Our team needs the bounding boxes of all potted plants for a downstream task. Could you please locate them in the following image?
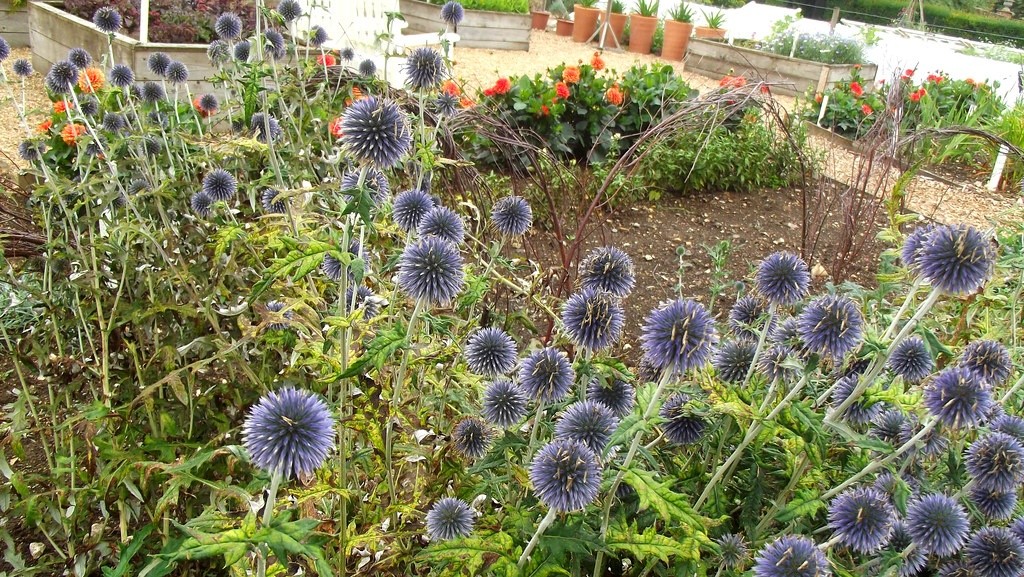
[661,0,695,61]
[694,8,727,41]
[628,0,659,54]
[397,0,531,53]
[573,0,601,44]
[598,0,629,48]
[28,0,340,136]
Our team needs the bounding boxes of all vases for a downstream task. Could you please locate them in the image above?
[531,10,550,31]
[556,19,574,38]
[682,36,879,104]
